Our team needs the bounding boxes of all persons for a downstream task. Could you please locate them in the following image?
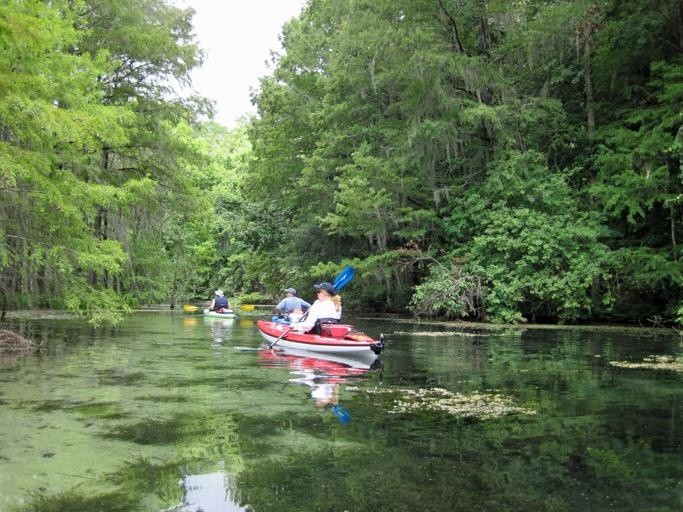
[209,290,231,311]
[295,373,333,408]
[274,282,342,334]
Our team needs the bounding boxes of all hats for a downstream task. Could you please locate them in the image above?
[284,287,297,295]
[313,282,337,295]
[215,290,224,297]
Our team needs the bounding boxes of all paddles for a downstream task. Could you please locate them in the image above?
[267,266,356,349]
[184,304,255,312]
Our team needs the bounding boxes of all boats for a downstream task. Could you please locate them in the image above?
[252,318,385,371]
[203,309,235,319]
[256,341,374,383]
